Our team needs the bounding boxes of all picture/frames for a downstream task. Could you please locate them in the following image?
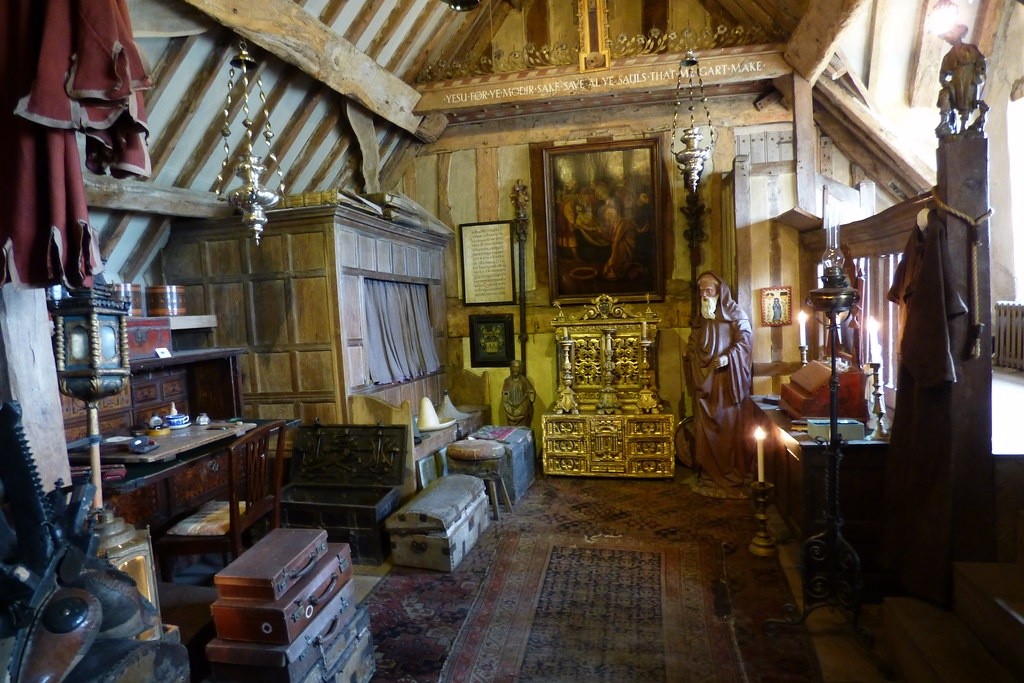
[542,137,665,306]
[468,313,515,368]
[460,220,517,306]
[761,286,792,326]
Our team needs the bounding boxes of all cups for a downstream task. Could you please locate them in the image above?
[166,414,189,425]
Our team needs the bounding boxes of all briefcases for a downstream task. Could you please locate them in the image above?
[206,527,376,683]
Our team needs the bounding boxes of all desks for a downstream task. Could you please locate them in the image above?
[750,394,890,546]
[68,418,300,524]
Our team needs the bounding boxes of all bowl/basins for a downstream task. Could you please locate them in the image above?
[145,286,186,316]
[108,284,142,316]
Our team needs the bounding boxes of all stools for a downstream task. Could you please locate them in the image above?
[447,455,513,521]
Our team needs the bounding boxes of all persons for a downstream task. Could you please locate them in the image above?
[682,271,753,488]
[502,360,536,426]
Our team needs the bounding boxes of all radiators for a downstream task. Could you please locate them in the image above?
[993,300,1024,371]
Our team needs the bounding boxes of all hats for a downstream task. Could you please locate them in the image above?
[436,390,474,420]
[414,397,456,431]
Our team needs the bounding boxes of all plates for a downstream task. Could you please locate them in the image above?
[170,421,191,429]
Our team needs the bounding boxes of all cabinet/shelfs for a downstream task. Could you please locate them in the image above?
[59,315,248,441]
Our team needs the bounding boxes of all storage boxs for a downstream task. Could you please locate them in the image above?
[279,417,408,566]
[807,418,865,440]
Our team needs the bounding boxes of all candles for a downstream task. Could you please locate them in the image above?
[751,425,767,482]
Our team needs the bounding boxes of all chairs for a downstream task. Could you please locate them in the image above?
[160,420,287,559]
